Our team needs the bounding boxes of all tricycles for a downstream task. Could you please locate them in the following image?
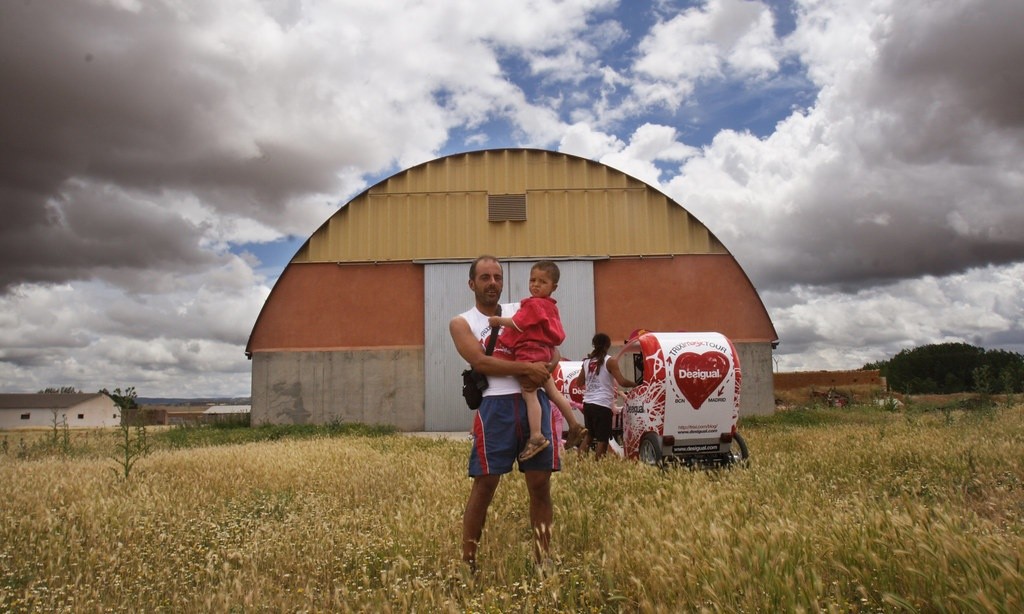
[604,332,750,474]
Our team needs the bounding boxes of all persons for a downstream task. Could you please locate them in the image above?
[576,333,637,461]
[449,256,566,579]
[488,260,588,461]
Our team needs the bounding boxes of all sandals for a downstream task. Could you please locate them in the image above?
[518,435,551,462]
[564,424,588,450]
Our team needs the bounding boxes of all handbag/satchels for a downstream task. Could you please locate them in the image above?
[461,368,489,411]
